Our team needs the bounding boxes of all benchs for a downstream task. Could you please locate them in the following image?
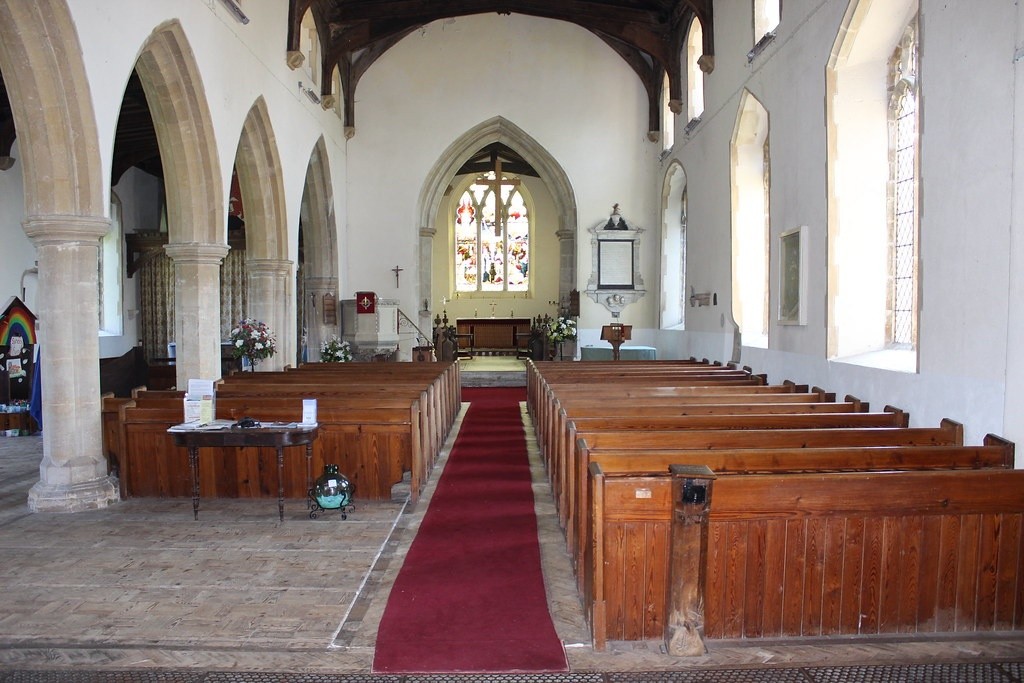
[526,357,1024,647]
[102,358,463,510]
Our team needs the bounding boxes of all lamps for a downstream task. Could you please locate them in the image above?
[746,31,777,63]
[683,116,701,135]
[303,87,321,105]
[221,0,250,25]
[656,148,671,163]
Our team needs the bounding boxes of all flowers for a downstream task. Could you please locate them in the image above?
[318,334,353,363]
[230,317,278,371]
[545,317,578,345]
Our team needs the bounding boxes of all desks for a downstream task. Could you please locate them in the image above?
[168,421,324,523]
[455,333,473,358]
[456,317,532,349]
[580,346,657,360]
[516,332,536,359]
